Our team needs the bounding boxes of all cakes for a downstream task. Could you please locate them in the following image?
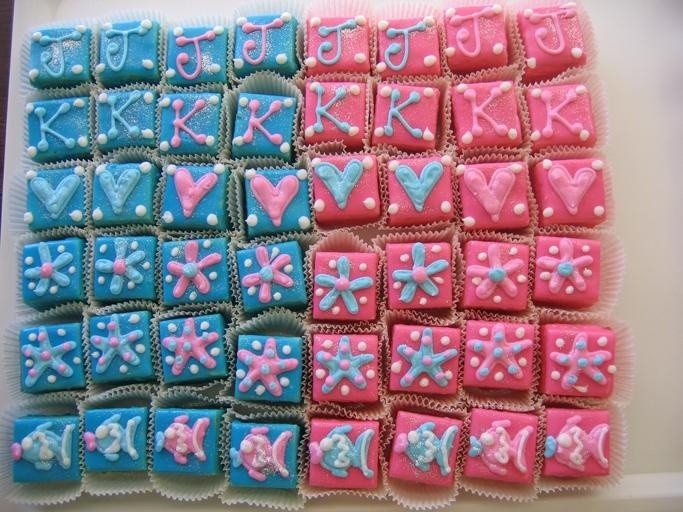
[456,161,530,232]
[23,165,86,232]
[93,235,158,303]
[91,161,159,226]
[459,240,530,314]
[450,79,523,152]
[89,310,156,385]
[84,406,148,472]
[464,319,535,390]
[158,92,222,156]
[231,92,297,162]
[523,85,597,153]
[234,333,304,404]
[303,81,367,148]
[158,314,228,386]
[235,240,309,313]
[160,163,230,232]
[312,332,379,404]
[162,236,231,306]
[18,322,86,394]
[303,15,371,76]
[22,237,86,312]
[444,4,509,73]
[518,2,587,84]
[311,154,380,225]
[165,24,229,86]
[531,158,608,226]
[233,12,299,77]
[152,408,225,476]
[95,18,159,87]
[534,235,601,308]
[375,15,441,79]
[307,418,380,490]
[243,167,311,239]
[96,89,157,154]
[27,24,91,87]
[386,241,453,310]
[229,420,300,489]
[25,94,90,164]
[386,154,454,226]
[387,411,463,488]
[389,323,462,396]
[539,323,618,399]
[10,414,81,483]
[371,82,441,151]
[312,251,379,322]
[465,408,539,484]
[542,406,611,478]
